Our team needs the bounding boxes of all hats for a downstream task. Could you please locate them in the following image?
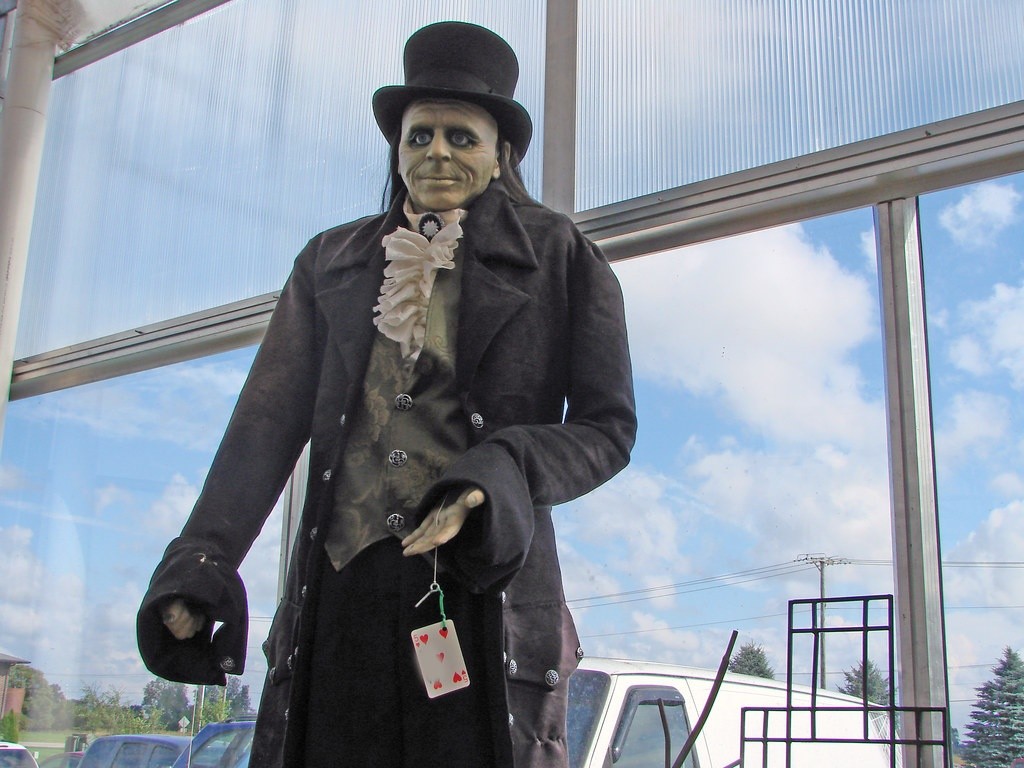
[372,22,533,168]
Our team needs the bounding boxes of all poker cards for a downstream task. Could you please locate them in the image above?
[410,619,471,699]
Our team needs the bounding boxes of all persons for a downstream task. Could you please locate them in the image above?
[136,21,638,768]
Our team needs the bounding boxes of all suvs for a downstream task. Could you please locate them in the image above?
[171,716,255,768]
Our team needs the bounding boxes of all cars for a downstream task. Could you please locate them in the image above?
[38,752,82,768]
[0,740,38,768]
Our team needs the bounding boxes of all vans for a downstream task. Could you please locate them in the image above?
[79,734,237,768]
[566,653,903,768]
